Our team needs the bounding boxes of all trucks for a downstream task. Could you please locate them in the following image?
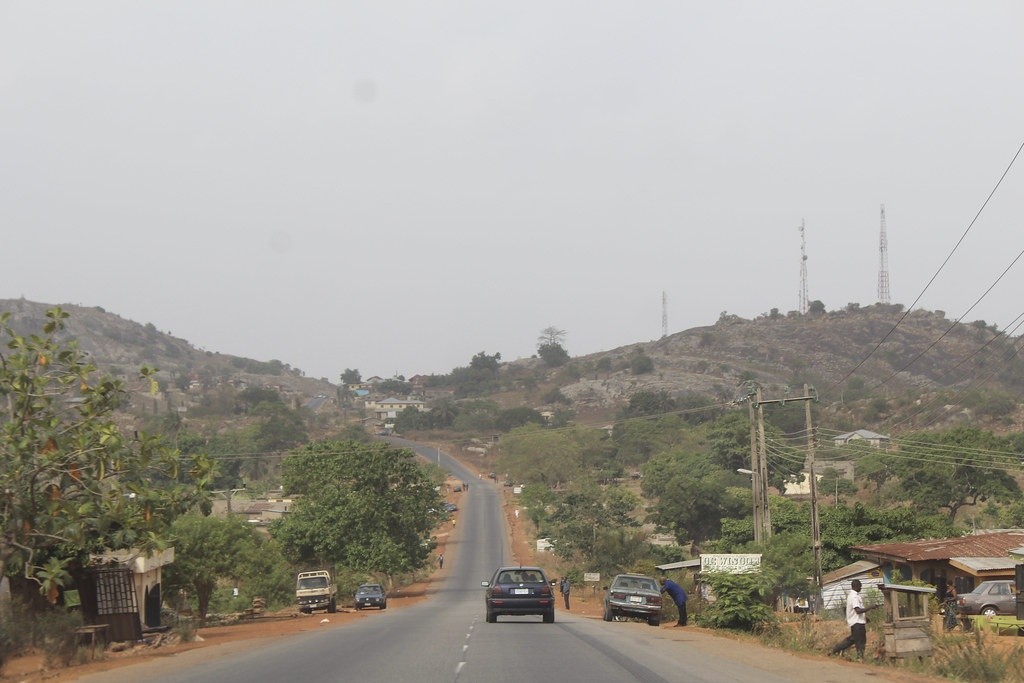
[296,570,339,614]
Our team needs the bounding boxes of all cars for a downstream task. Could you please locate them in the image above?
[481,566,558,624]
[602,574,663,627]
[354,584,387,610]
[956,580,1017,615]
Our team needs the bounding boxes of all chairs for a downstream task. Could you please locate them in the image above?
[529,574,537,580]
[505,578,514,583]
[620,581,628,586]
[641,583,651,589]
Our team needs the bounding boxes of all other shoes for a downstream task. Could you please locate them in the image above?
[674,621,686,627]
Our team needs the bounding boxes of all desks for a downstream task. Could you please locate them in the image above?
[76,623,110,660]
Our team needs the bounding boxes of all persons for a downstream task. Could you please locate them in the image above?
[943,580,957,630]
[660,577,688,627]
[452,519,456,527]
[479,473,481,479]
[831,580,877,660]
[462,483,468,491]
[560,577,570,610]
[437,554,443,569]
[521,573,528,581]
[494,473,496,481]
[515,509,519,518]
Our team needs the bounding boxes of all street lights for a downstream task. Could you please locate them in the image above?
[737,469,771,536]
